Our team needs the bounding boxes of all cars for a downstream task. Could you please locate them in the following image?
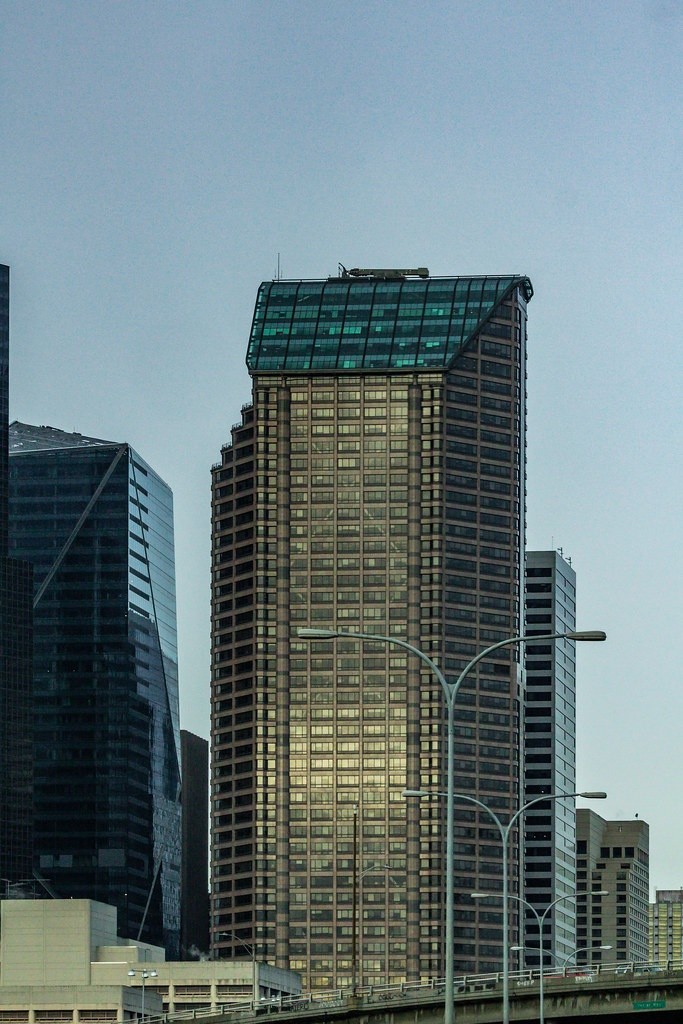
[616,963,662,974]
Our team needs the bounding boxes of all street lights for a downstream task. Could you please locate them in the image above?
[400,788,609,1024]
[470,890,610,1024]
[217,931,256,1001]
[350,803,396,996]
[296,624,610,1024]
[128,968,159,1022]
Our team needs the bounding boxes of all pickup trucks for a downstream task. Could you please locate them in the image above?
[543,966,595,979]
